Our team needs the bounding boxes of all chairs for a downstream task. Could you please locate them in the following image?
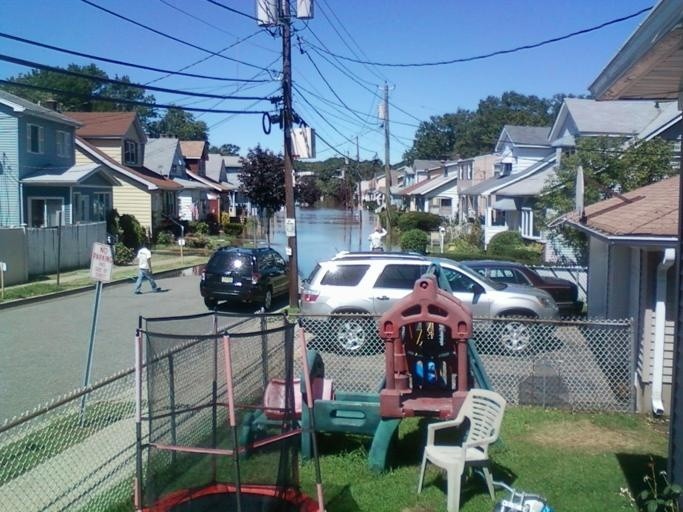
[417,387,507,512]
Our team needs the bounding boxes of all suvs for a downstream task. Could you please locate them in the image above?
[297,252,562,357]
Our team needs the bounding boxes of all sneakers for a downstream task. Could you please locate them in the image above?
[157,288,161,292]
[134,292,142,294]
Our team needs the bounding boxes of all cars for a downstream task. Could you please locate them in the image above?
[199,246,295,311]
[459,260,585,314]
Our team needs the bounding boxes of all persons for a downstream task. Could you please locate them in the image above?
[132,241,160,293]
[367,225,388,254]
